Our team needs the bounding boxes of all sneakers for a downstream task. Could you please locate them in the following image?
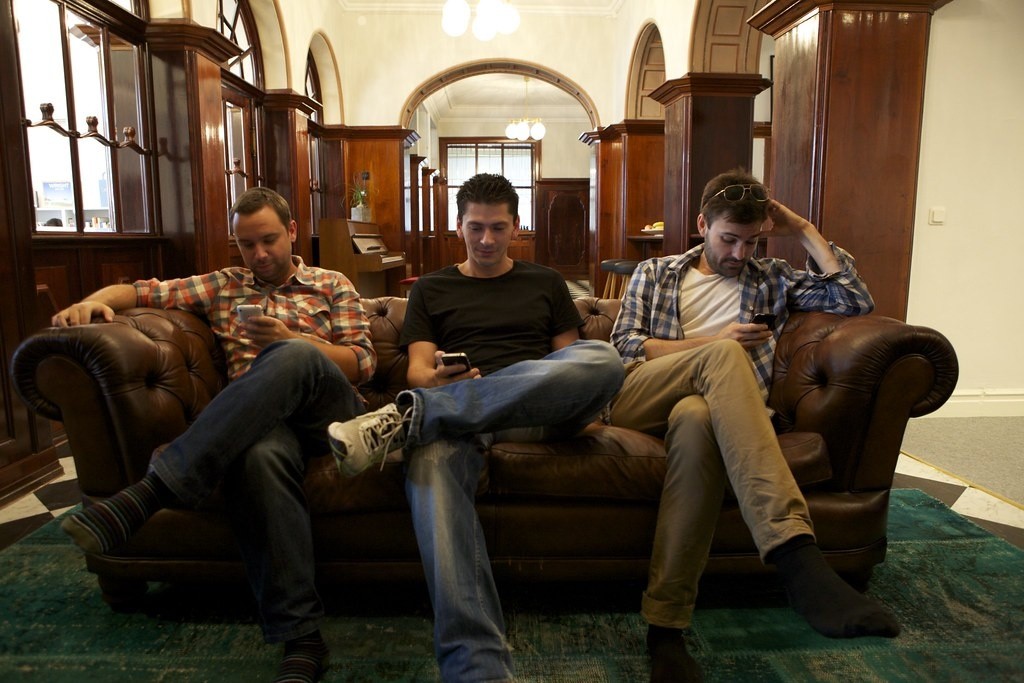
[327,402,404,481]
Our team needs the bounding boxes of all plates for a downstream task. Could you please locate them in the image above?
[641,229,664,236]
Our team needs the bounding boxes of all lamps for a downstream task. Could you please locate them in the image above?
[440,0,521,42]
[504,76,546,141]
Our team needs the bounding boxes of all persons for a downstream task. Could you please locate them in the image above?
[50,187,378,682]
[597,166,901,682]
[327,171,626,683]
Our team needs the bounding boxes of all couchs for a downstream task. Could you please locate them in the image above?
[10,294,960,639]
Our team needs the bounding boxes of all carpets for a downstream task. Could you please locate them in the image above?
[0,483,1024,682]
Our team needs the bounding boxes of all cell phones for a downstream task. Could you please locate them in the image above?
[750,313,777,330]
[237,305,264,323]
[442,353,471,377]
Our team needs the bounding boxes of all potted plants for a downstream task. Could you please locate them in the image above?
[338,170,372,223]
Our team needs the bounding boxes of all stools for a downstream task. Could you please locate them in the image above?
[600,259,629,299]
[612,260,639,299]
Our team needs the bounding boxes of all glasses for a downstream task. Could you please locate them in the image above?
[700,183,770,212]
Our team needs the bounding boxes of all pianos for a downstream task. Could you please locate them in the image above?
[318,216,405,299]
